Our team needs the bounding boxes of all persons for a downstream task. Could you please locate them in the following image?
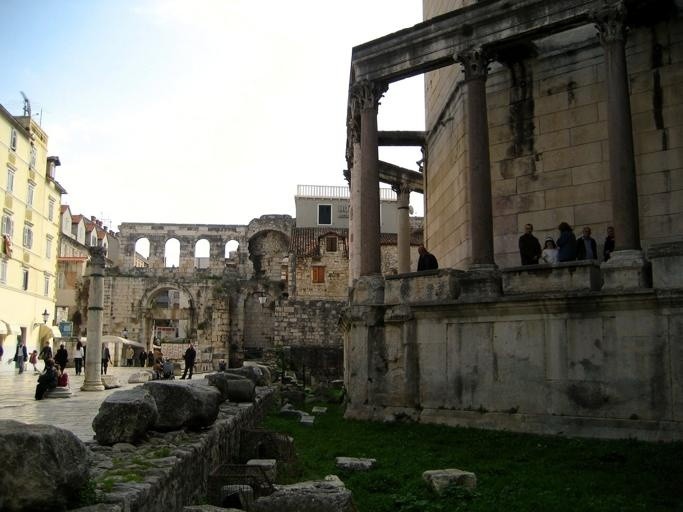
[0,340,174,400]
[576,227,597,260]
[518,223,542,264]
[541,236,559,263]
[555,222,578,261]
[415,246,438,270]
[601,226,616,260]
[179,343,195,380]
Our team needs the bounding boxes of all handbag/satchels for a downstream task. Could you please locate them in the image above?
[57,374,67,387]
